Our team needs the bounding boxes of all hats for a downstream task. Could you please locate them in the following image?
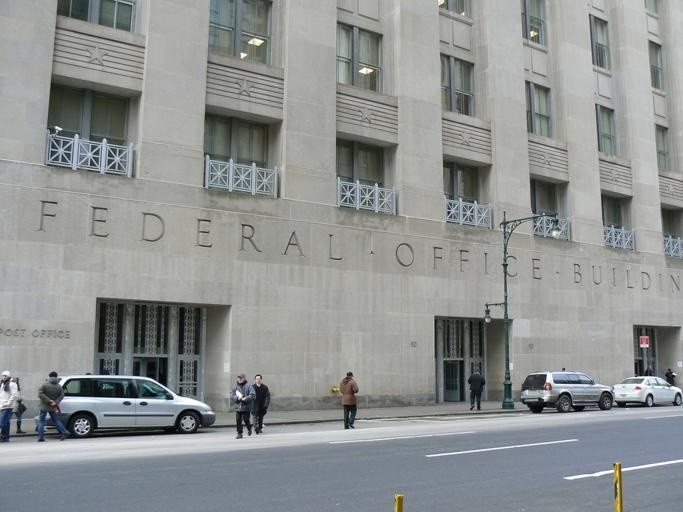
[237,372,246,378]
[1,370,12,378]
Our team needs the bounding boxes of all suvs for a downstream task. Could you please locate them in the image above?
[519,368,616,415]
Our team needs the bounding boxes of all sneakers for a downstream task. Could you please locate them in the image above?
[344,422,355,430]
[469,404,481,410]
[36,437,46,442]
[60,431,71,441]
[235,427,263,439]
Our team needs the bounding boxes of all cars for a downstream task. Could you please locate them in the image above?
[612,375,682,408]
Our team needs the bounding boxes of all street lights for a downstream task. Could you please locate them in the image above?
[482,210,562,411]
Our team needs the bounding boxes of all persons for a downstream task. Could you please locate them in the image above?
[0,370,17,442]
[8,373,27,433]
[466,367,485,411]
[664,367,673,387]
[339,370,358,429]
[36,370,71,441]
[249,374,270,435]
[644,364,653,377]
[228,372,256,439]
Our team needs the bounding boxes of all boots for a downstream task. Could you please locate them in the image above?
[16,420,27,434]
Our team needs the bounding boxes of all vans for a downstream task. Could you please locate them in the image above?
[33,374,215,437]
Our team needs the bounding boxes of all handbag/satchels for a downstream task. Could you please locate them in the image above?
[14,400,28,419]
